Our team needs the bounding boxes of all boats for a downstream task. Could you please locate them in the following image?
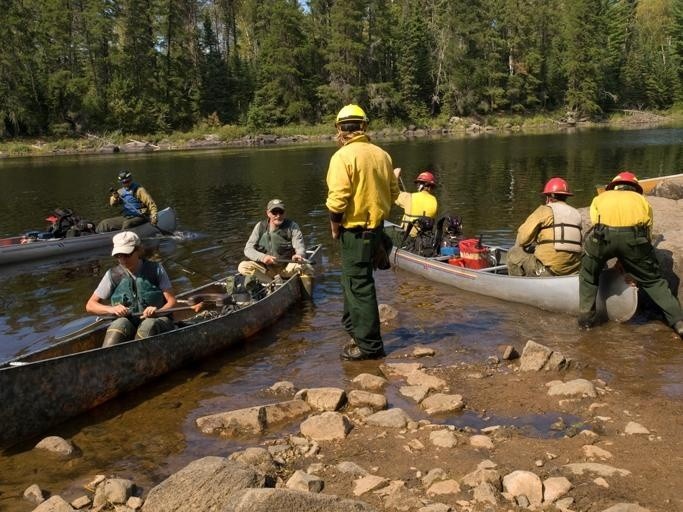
[381,219,638,322]
[0,206,176,267]
[0,242,329,452]
[595,173,682,196]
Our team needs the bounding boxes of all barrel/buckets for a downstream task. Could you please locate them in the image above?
[458,238,490,271]
[447,258,466,269]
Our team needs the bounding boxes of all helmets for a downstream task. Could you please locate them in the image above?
[335,104,369,125]
[118,171,133,183]
[413,172,438,186]
[541,178,574,196]
[606,171,643,193]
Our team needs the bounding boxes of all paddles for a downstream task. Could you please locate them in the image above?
[56,302,205,338]
[108,186,174,236]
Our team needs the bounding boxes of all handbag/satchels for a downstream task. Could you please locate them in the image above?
[374,230,393,270]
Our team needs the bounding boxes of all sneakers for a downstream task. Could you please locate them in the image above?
[340,344,366,360]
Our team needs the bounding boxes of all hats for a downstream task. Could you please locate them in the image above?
[111,231,143,256]
[266,198,285,211]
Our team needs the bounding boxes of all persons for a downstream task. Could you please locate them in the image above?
[578,171,683,337]
[506,177,583,277]
[85,231,178,348]
[383,168,439,250]
[97,169,158,232]
[238,199,316,310]
[326,103,400,361]
